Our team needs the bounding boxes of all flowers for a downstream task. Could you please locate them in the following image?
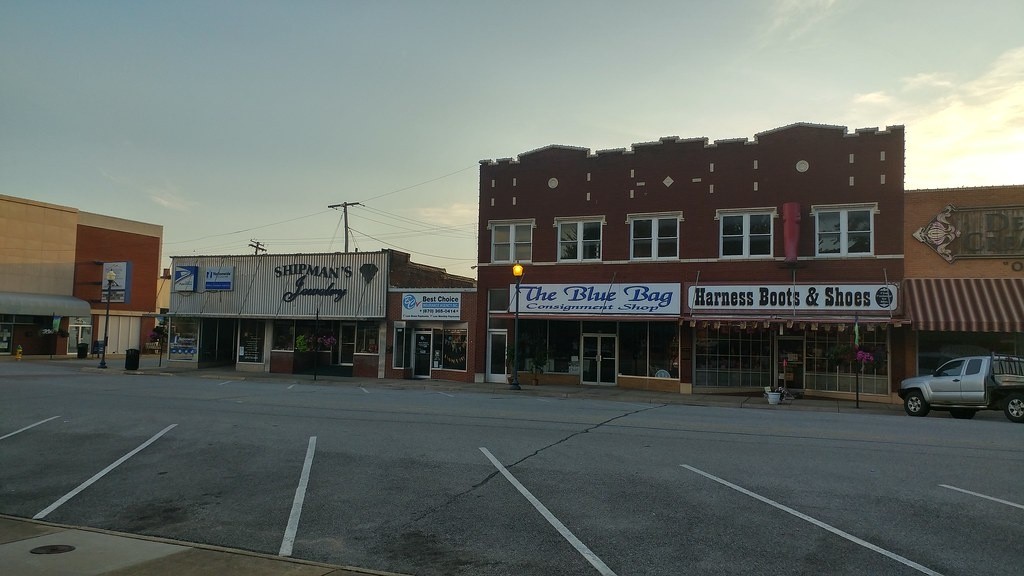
[762,387,787,401]
[318,333,337,348]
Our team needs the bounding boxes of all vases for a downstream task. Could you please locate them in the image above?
[767,392,781,405]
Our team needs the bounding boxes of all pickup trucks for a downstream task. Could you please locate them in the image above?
[898,355,1024,423]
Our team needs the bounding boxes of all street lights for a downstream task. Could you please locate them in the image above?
[98,269,116,368]
[509,260,523,390]
